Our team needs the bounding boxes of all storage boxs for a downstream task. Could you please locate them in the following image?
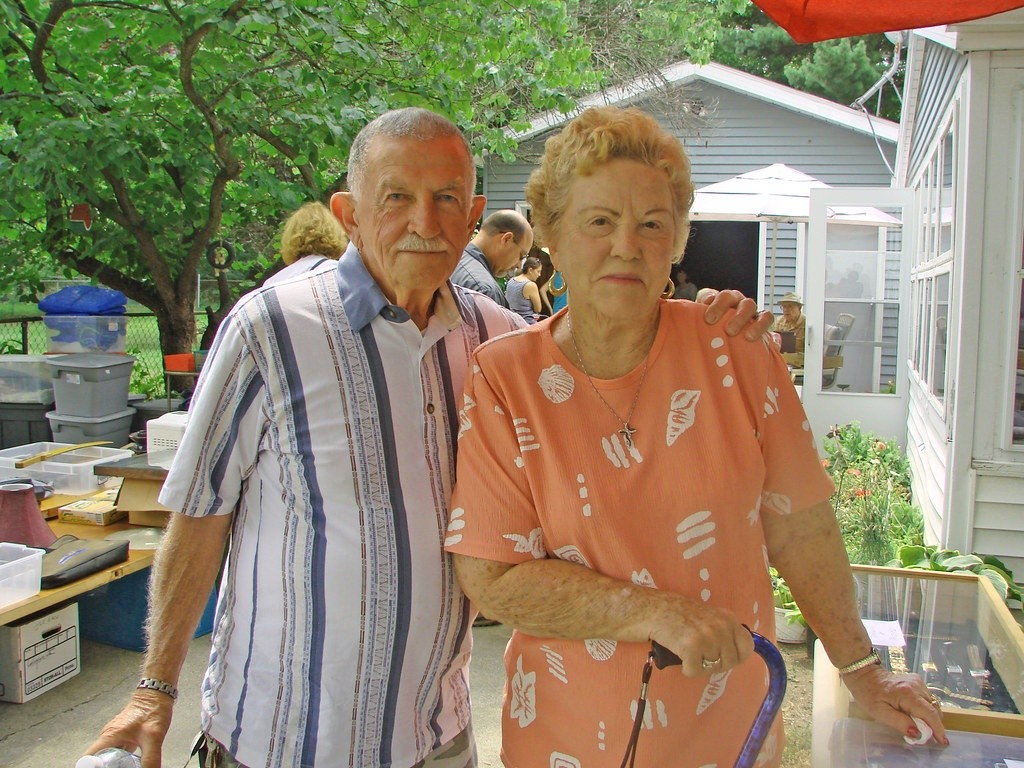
[0,601,81,704]
[0,542,46,609]
[67,567,217,653]
[0,285,208,527]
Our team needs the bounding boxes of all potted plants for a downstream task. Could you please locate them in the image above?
[769,567,808,643]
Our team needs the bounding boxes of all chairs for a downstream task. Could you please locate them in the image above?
[790,314,854,393]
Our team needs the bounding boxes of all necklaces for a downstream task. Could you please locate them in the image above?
[566,312,655,446]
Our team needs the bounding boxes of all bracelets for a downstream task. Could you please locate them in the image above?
[137,678,179,700]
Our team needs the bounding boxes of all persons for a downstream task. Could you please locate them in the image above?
[505,256,543,325]
[262,202,347,289]
[89,106,773,768]
[449,209,534,310]
[540,270,568,315]
[443,105,949,768]
[773,291,807,368]
[674,268,697,302]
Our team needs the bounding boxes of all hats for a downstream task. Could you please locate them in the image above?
[777,292,805,306]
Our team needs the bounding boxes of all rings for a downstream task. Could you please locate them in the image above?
[703,658,720,668]
[930,694,941,707]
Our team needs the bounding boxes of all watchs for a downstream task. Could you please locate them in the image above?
[839,646,882,673]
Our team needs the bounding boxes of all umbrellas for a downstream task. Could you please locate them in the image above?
[688,164,902,313]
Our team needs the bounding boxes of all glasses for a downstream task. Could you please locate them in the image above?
[534,260,541,266]
[780,305,795,310]
[499,231,529,259]
